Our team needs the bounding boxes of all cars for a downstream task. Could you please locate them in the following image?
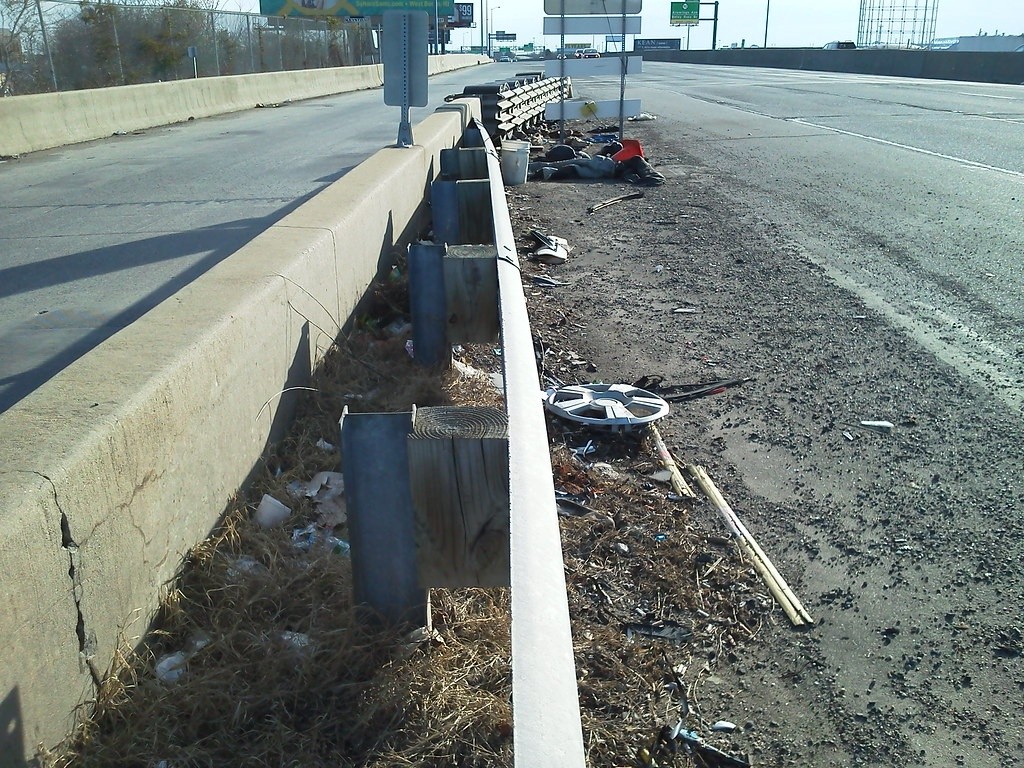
[574,48,584,58]
[823,41,857,50]
[556,54,567,60]
[582,47,600,58]
[499,55,511,62]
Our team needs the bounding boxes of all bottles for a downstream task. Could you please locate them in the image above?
[326,537,349,557]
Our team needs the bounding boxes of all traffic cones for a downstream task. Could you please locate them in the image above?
[610,138,644,162]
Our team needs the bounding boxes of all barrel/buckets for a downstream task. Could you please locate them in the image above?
[501,140,530,186]
